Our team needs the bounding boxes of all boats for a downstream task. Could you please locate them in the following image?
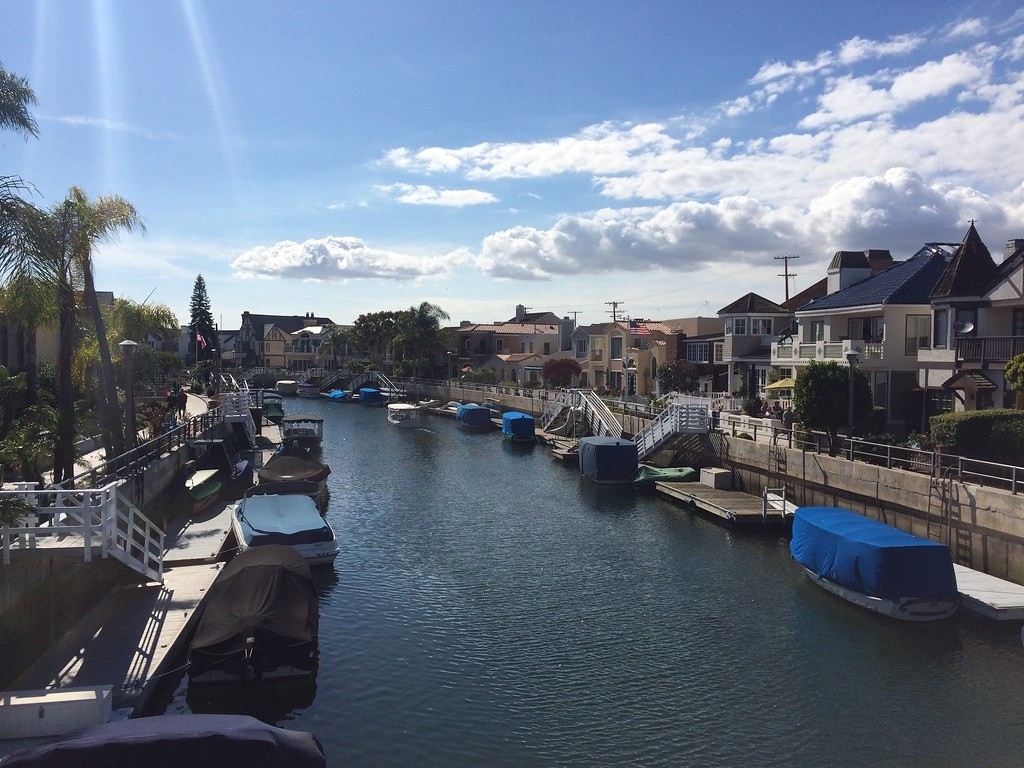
[386,403,421,428]
[632,465,698,489]
[788,505,959,623]
[257,455,332,498]
[419,399,443,411]
[480,397,500,419]
[501,411,536,443]
[183,542,320,700]
[231,480,341,570]
[324,389,351,402]
[578,436,639,485]
[281,413,325,448]
[262,391,284,424]
[296,383,320,398]
[359,387,381,405]
[455,405,491,428]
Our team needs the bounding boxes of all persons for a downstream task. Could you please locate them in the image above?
[773,401,784,420]
[166,391,177,418]
[782,408,797,429]
[760,400,769,418]
[178,389,187,419]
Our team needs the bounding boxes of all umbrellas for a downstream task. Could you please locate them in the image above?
[762,377,797,406]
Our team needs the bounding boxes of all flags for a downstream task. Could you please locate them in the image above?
[628,319,651,336]
[196,327,207,350]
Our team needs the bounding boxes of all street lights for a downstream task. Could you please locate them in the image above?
[844,349,860,460]
[447,351,452,388]
[210,348,217,396]
[117,339,138,477]
